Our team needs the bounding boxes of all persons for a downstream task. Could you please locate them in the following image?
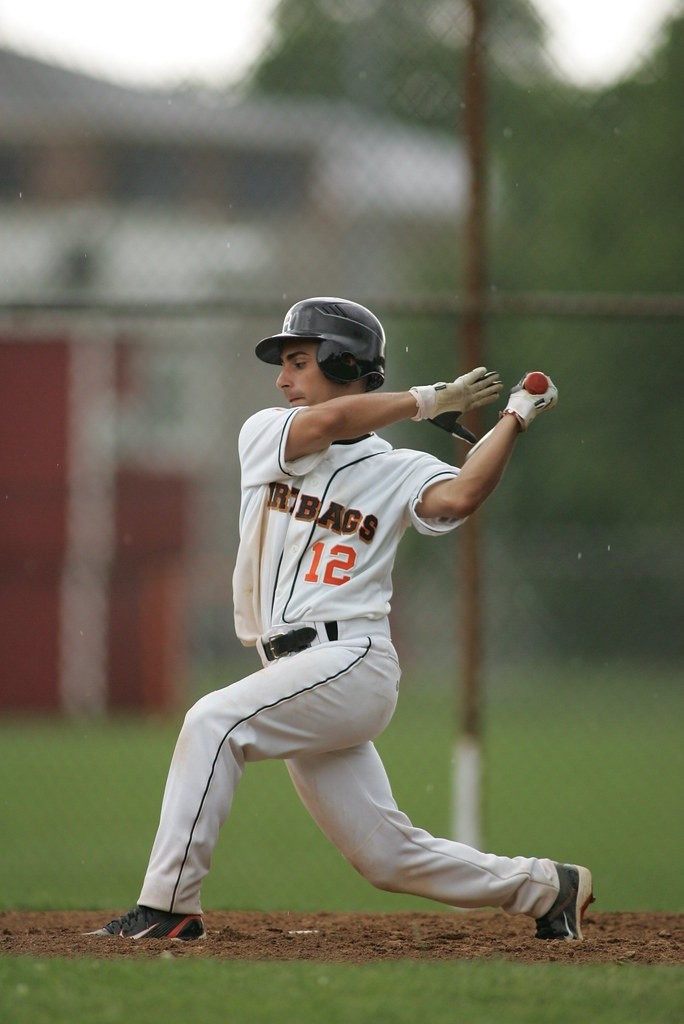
[82,295,594,939]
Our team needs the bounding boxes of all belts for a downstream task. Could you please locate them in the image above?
[263,621,338,661]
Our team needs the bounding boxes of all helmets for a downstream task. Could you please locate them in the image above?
[255,296,386,393]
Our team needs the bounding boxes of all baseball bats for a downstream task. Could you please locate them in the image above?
[523,370,550,396]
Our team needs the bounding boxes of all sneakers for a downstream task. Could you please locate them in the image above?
[81,904,205,940]
[535,861,591,941]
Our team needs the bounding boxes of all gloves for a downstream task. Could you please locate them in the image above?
[409,367,504,445]
[503,371,558,433]
